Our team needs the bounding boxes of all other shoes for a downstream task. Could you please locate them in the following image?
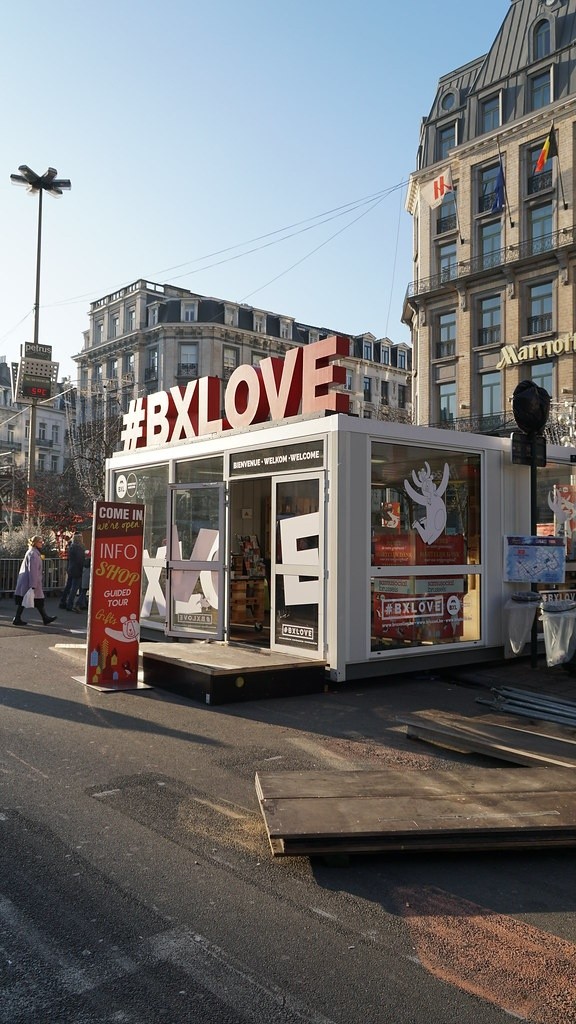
[12,617,28,625]
[72,606,83,613]
[59,603,68,609]
[43,615,57,625]
[66,606,73,611]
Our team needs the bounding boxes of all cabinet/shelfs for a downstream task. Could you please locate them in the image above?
[229,580,265,632]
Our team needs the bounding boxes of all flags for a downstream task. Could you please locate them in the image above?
[421,167,454,210]
[535,124,559,172]
[490,166,504,212]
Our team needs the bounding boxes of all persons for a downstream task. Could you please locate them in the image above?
[58,531,91,614]
[11,534,58,625]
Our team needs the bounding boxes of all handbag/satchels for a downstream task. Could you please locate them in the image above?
[22,587,35,608]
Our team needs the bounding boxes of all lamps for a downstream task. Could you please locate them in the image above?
[458,261,465,267]
[560,228,568,235]
[508,397,513,402]
[507,245,514,251]
[561,389,569,394]
[459,404,467,410]
